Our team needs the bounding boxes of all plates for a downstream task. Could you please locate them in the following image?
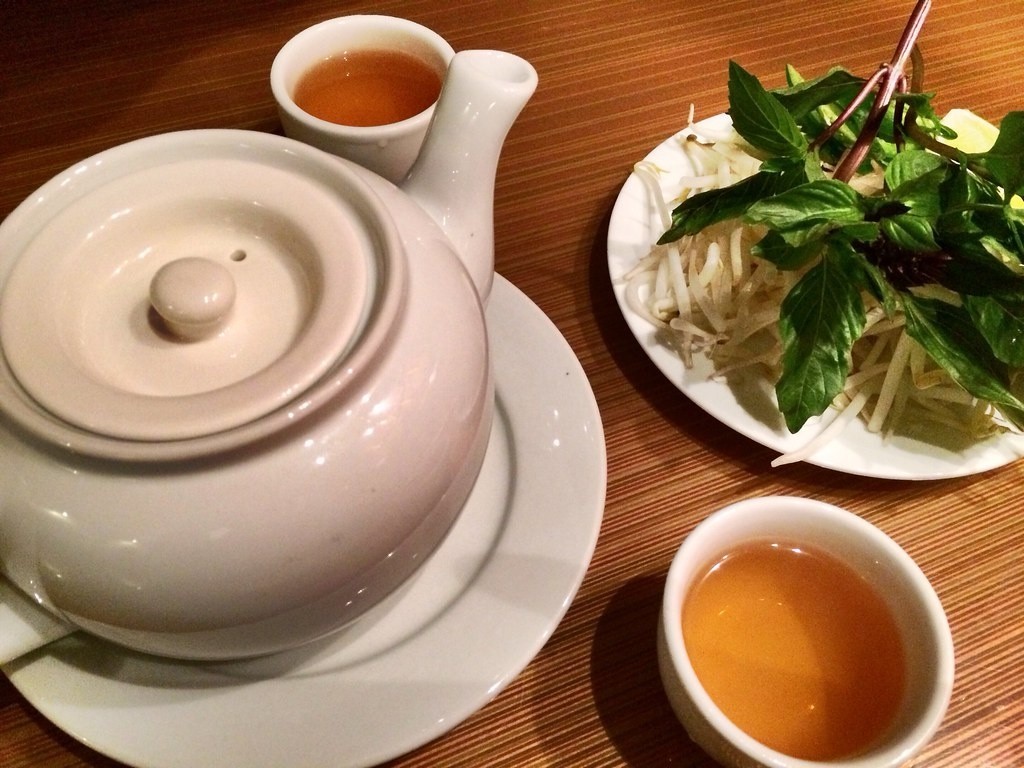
[606,112,1024,480]
[1,265,607,767]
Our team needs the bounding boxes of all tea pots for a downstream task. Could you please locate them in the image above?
[0,49,535,663]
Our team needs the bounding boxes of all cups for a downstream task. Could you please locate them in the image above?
[268,15,456,187]
[658,495,954,768]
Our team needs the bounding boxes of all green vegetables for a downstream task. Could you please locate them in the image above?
[655,57,1024,435]
